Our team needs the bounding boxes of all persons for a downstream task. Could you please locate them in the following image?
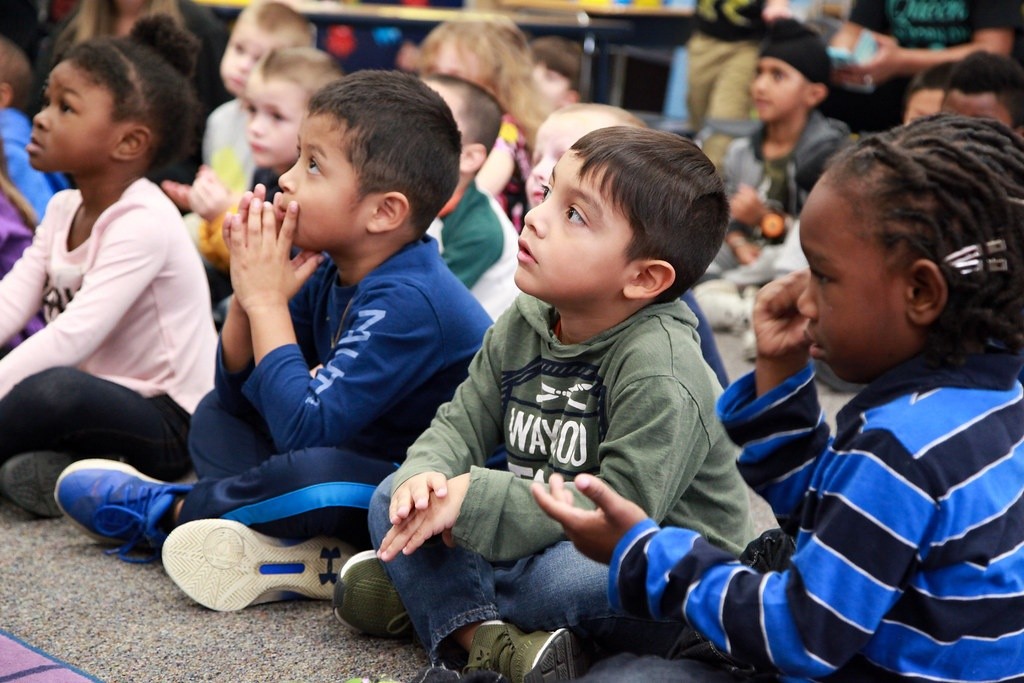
[528,114,1023,683]
[330,123,751,683]
[53,68,496,614]
[0,13,221,518]
[0,1,1024,388]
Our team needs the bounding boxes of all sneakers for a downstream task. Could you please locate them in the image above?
[161,517,359,612]
[54,457,194,564]
[331,547,424,649]
[0,450,134,520]
[460,617,589,683]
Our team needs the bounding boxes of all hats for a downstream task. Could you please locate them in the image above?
[756,17,834,86]
